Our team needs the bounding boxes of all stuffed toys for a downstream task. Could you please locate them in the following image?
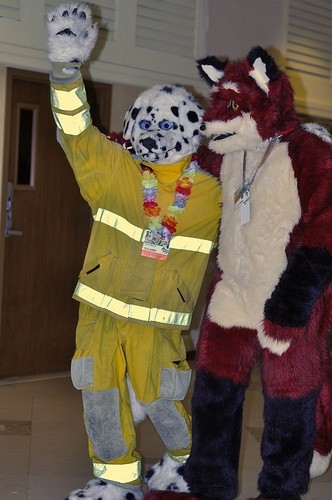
[303,119,330,478]
[105,48,330,500]
[43,1,222,499]
